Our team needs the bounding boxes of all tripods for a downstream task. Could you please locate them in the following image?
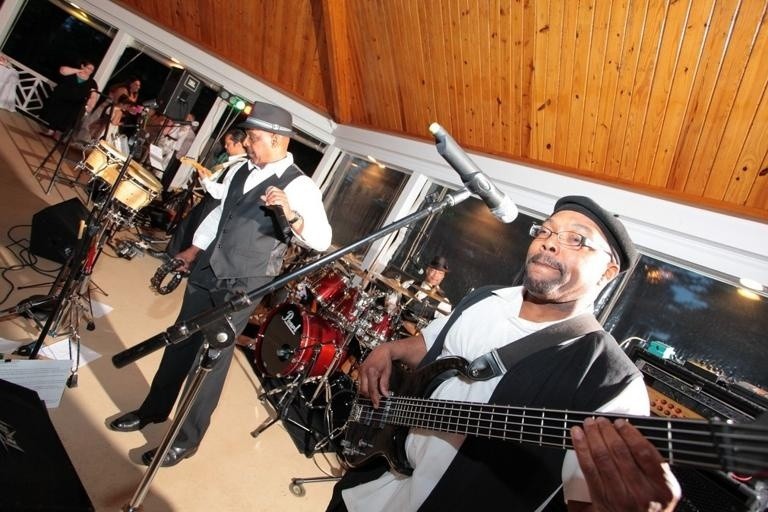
[0,208,95,390]
[147,150,197,235]
[259,296,379,452]
[254,349,319,439]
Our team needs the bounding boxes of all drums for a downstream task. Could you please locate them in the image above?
[102,159,162,211]
[304,261,351,307]
[256,303,347,383]
[358,301,397,347]
[84,140,125,185]
[327,284,369,329]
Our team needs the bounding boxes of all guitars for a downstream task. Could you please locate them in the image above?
[180,155,213,177]
[341,355,767,485]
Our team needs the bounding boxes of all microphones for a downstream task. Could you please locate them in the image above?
[430,123,519,224]
[177,119,199,129]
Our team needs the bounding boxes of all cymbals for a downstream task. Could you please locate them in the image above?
[331,246,366,273]
[410,284,452,304]
[372,271,421,303]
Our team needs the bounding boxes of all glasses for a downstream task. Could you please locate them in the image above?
[528,223,613,264]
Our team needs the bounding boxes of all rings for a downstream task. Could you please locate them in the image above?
[647,502,660,511]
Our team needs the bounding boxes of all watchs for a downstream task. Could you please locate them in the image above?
[288,210,301,225]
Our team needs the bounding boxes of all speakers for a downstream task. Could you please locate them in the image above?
[157,65,206,120]
[34,197,104,263]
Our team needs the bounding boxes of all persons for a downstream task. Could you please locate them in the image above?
[70,78,197,192]
[38,60,98,141]
[109,101,333,467]
[144,129,250,278]
[324,194,684,512]
[387,255,452,324]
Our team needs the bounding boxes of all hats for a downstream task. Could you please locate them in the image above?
[239,101,294,138]
[551,195,637,273]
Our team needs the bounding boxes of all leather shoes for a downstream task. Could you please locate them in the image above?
[111,409,165,433]
[140,440,199,467]
